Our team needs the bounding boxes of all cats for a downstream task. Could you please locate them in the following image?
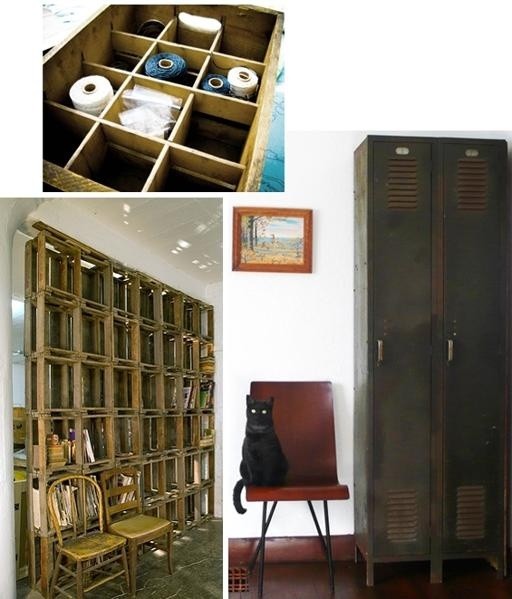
[233,394,288,515]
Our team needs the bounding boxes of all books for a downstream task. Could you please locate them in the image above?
[199,413,213,516]
[169,338,216,411]
[14,426,143,536]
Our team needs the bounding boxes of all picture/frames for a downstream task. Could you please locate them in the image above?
[230,205,313,274]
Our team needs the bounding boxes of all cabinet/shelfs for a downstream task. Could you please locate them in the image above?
[24,220,215,599]
[352,136,511,587]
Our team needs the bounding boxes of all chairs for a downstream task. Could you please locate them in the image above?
[47,467,174,598]
[245,381,350,598]
